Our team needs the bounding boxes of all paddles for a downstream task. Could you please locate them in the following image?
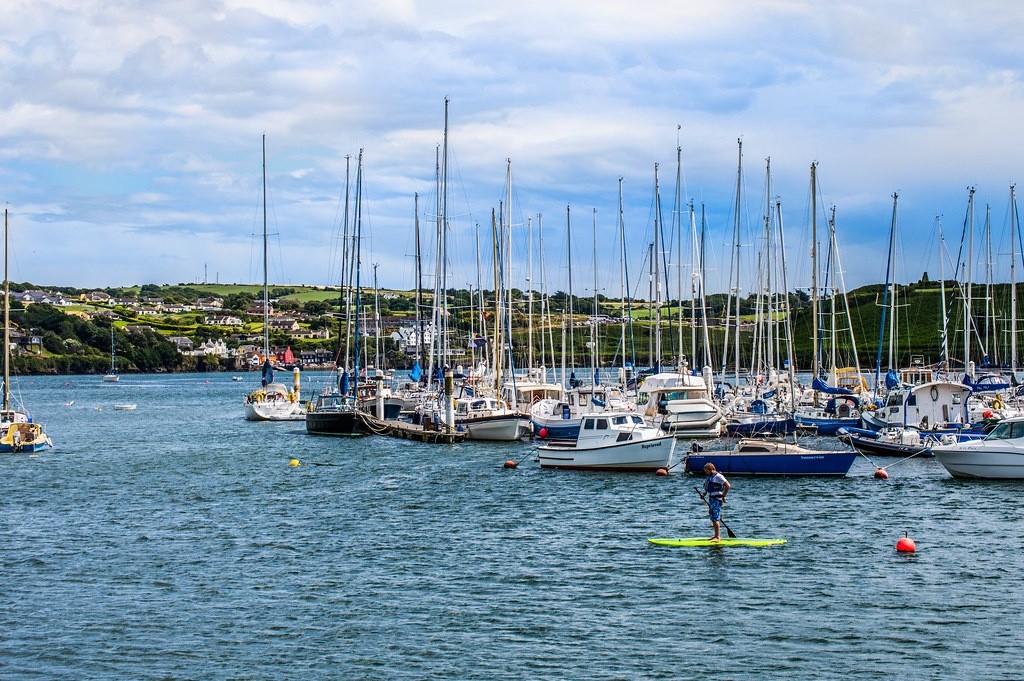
[693,486,737,538]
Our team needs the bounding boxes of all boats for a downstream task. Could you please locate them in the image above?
[114,405,138,411]
[103,373,119,383]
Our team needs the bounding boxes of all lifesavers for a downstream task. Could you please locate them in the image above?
[993,399,1002,410]
[255,393,263,402]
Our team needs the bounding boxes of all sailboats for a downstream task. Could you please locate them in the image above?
[0,210,53,452]
[244,134,311,421]
[306,97,1024,481]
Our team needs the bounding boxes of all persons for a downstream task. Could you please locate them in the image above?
[700,463,730,541]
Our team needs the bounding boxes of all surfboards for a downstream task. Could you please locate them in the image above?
[646,537,788,546]
